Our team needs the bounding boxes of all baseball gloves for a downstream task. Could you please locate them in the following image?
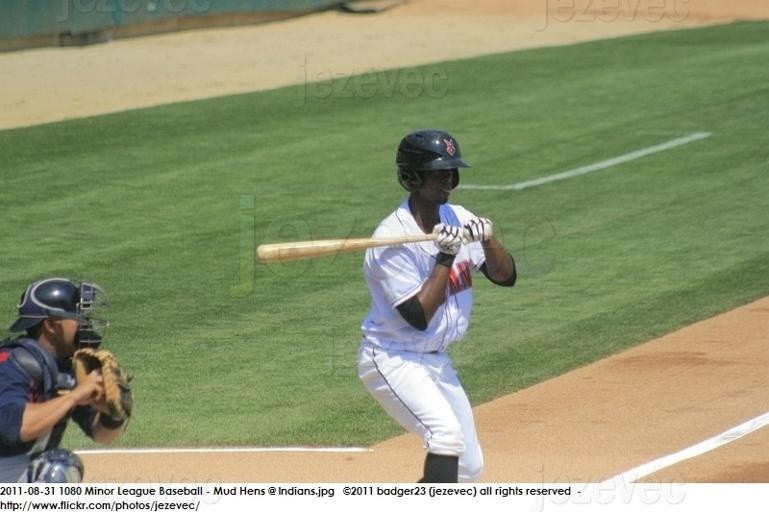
[73,348,134,422]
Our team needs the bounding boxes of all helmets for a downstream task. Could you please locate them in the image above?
[27,448,84,483]
[396,130,471,192]
[10,278,108,348]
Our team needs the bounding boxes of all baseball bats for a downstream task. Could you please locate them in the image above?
[256,230,471,260]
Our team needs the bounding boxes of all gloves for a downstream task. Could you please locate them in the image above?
[433,217,492,256]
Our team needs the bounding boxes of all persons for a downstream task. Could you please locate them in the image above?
[0,276,130,481]
[356,130,517,483]
